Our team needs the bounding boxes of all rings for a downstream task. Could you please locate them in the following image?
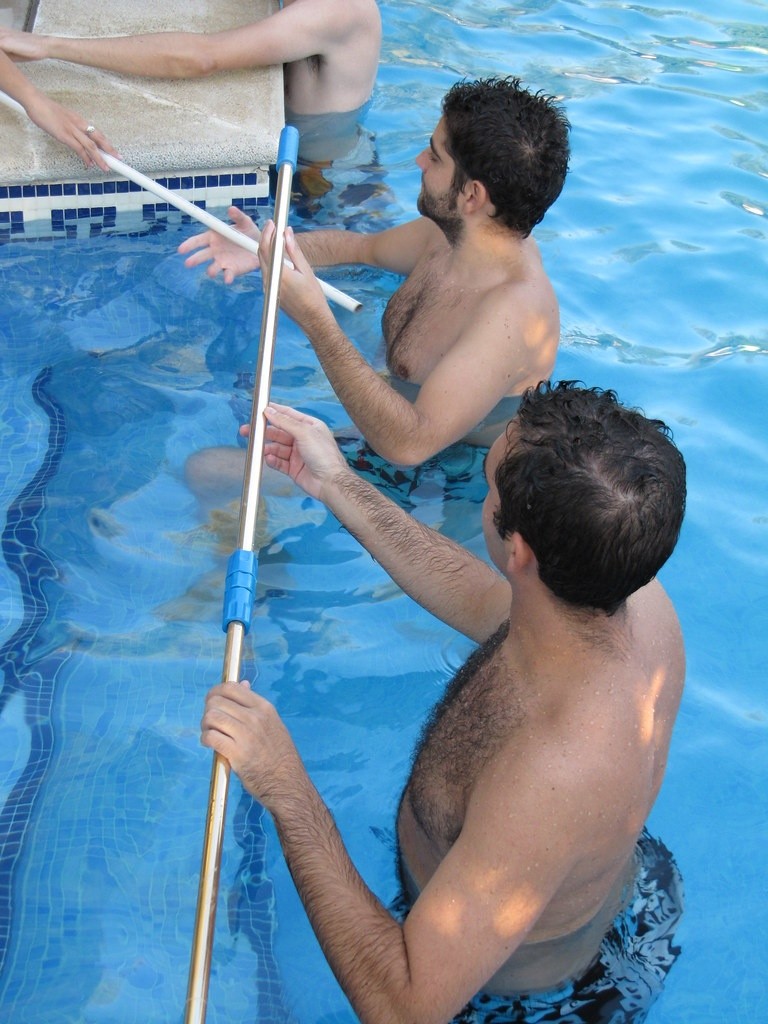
[86,125,96,134]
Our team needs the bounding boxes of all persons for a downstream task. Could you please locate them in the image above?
[0,0,384,171]
[176,75,573,468]
[201,381,688,1023]
